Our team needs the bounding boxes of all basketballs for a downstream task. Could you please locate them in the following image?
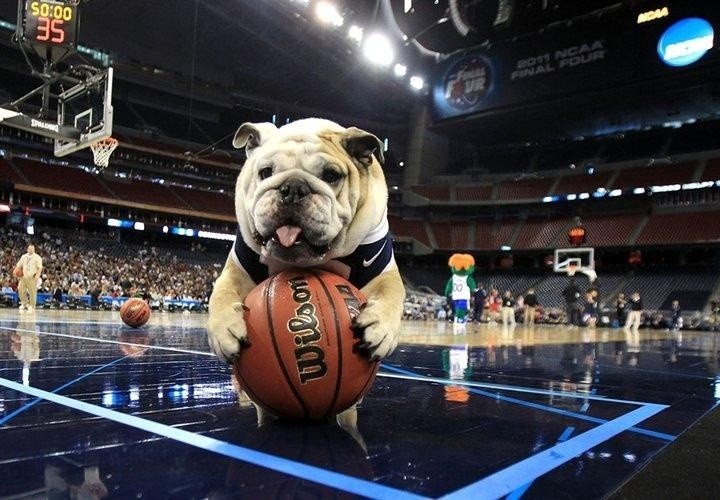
[120,298,150,327]
[232,268,380,422]
[12,268,23,278]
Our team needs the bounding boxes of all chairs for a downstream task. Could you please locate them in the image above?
[388,161,716,311]
[0,126,236,266]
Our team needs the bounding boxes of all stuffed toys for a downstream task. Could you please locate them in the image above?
[445,253,475,325]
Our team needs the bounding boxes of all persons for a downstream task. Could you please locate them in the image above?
[469,278,720,330]
[522,346,537,369]
[582,331,594,368]
[12,311,41,371]
[615,330,626,366]
[0,213,220,311]
[623,330,641,366]
[405,294,454,323]
[669,330,683,364]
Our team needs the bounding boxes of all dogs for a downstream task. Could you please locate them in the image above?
[205,115,407,402]
